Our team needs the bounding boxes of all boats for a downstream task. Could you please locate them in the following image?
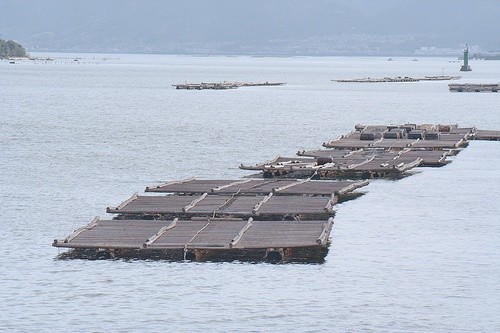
[28,56,54,60]
